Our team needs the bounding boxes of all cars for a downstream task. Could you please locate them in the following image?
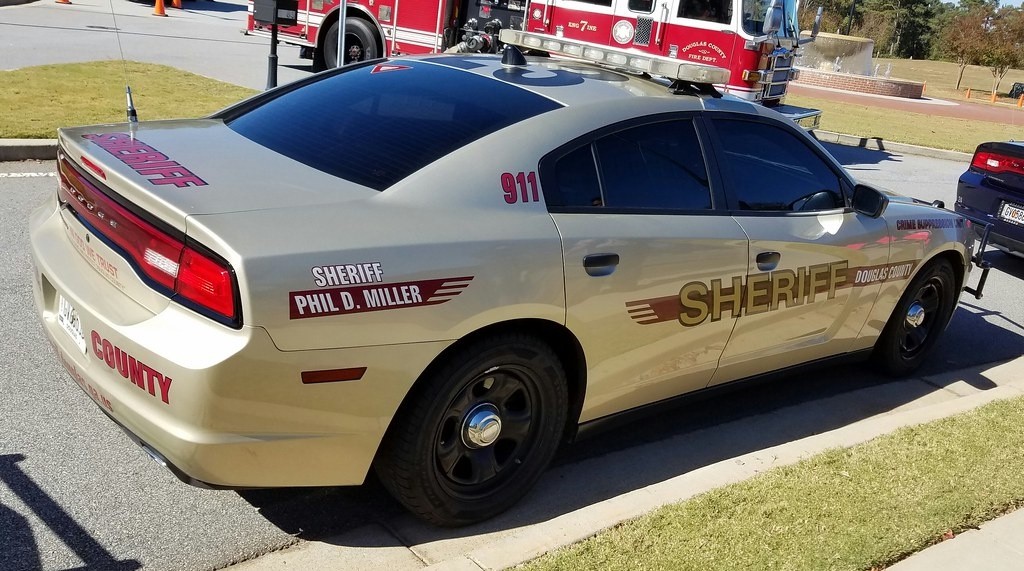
[27,47,976,529]
[954,139,1024,260]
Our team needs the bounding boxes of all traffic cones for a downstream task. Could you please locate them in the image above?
[56,0,72,4]
[170,0,184,9]
[152,0,168,17]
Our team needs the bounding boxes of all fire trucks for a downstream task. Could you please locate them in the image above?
[245,0,824,133]
